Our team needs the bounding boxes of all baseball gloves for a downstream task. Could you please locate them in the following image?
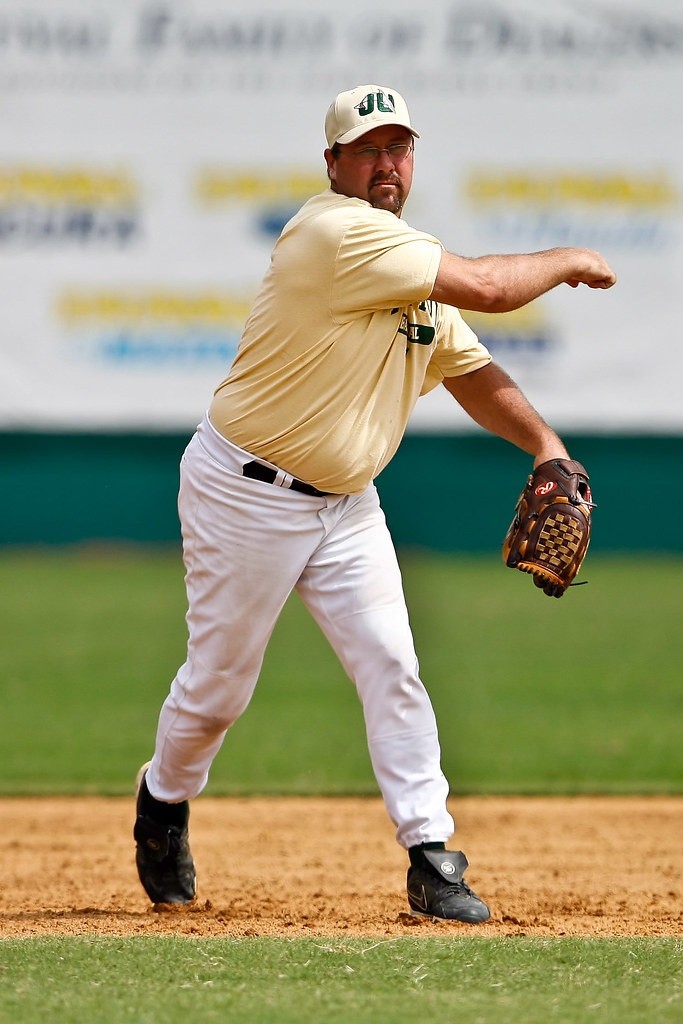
[502,457,598,599]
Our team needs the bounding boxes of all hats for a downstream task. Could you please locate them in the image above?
[325,84,421,149]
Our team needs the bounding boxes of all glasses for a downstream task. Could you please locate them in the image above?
[337,144,413,159]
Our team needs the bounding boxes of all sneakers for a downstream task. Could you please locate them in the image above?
[407,842,490,923]
[133,760,198,905]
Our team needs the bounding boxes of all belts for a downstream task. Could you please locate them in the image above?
[242,460,343,497]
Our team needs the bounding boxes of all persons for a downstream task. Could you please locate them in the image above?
[132,86,616,925]
